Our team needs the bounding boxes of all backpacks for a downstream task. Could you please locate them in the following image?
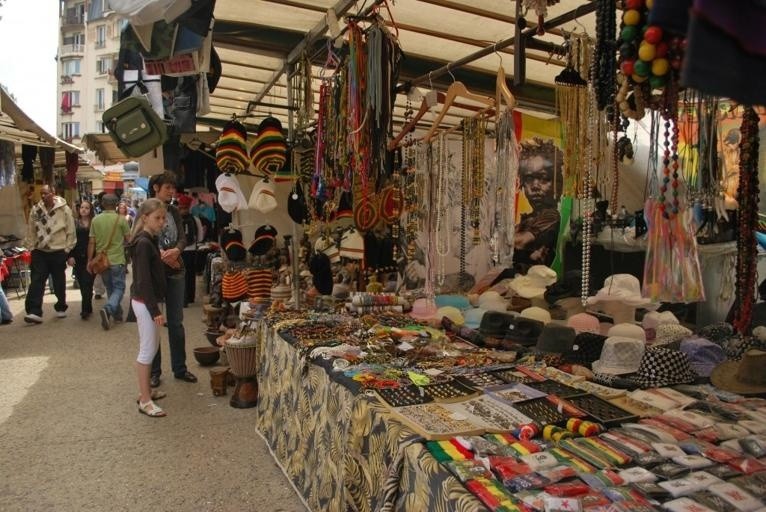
[180,213,199,247]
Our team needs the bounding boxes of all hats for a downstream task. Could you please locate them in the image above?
[218,269,250,304]
[248,116,289,176]
[214,120,252,175]
[508,262,559,300]
[216,226,248,263]
[625,346,697,388]
[679,336,729,378]
[543,272,602,302]
[696,320,736,345]
[558,331,609,363]
[177,194,193,209]
[213,172,250,214]
[474,310,516,335]
[313,235,342,264]
[515,305,553,325]
[460,308,485,331]
[584,272,652,307]
[247,267,275,306]
[709,347,766,396]
[473,290,511,306]
[590,338,647,376]
[606,322,648,349]
[408,296,439,322]
[649,322,694,348]
[718,335,765,361]
[429,305,465,327]
[496,316,546,341]
[525,325,577,354]
[338,227,366,260]
[247,177,279,214]
[472,299,521,318]
[565,312,602,336]
[434,293,471,308]
[248,224,280,259]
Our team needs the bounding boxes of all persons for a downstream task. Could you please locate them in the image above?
[509,136,562,266]
[1,170,203,417]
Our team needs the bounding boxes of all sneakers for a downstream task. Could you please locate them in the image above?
[113,315,123,325]
[98,306,112,331]
[56,310,67,319]
[23,312,44,325]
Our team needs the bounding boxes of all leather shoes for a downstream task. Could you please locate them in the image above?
[149,375,160,388]
[173,371,198,384]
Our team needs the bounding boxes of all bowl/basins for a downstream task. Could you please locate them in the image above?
[193,347,220,364]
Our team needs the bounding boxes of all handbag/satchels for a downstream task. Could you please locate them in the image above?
[171,95,197,130]
[88,251,110,274]
[101,95,170,159]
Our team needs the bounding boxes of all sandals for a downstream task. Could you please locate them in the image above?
[136,390,168,404]
[137,399,168,418]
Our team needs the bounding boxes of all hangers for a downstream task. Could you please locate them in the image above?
[345,11,382,36]
[387,71,496,152]
[319,38,341,79]
[494,40,519,126]
[424,63,496,144]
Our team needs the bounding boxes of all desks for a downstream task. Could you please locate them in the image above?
[574,234,766,335]
[257,313,765,511]
